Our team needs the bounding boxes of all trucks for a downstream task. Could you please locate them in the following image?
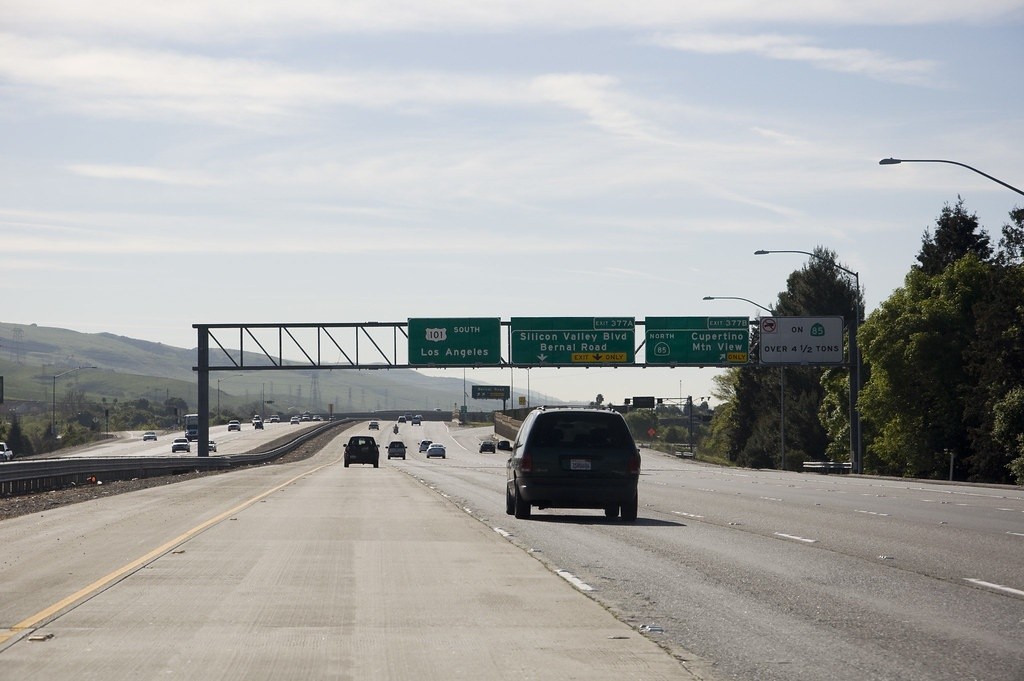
[183,413,198,441]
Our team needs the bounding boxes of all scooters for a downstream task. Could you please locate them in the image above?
[393,426,399,434]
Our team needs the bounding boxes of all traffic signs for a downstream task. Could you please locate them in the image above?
[407,317,501,366]
[645,316,749,365]
[510,316,635,367]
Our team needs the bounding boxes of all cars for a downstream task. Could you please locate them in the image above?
[478,440,496,454]
[143,431,157,441]
[417,440,434,453]
[290,416,299,425]
[0,441,13,461]
[426,443,447,459]
[208,440,216,452]
[398,416,406,423]
[171,437,190,452]
[405,412,424,426]
[368,421,380,431]
[385,440,408,460]
[498,440,510,450]
[252,413,337,431]
[228,420,241,431]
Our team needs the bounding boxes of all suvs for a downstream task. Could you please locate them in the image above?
[496,404,642,523]
[343,435,381,468]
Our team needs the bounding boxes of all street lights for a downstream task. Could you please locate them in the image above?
[703,297,786,470]
[52,365,98,438]
[217,374,243,424]
[754,250,861,474]
